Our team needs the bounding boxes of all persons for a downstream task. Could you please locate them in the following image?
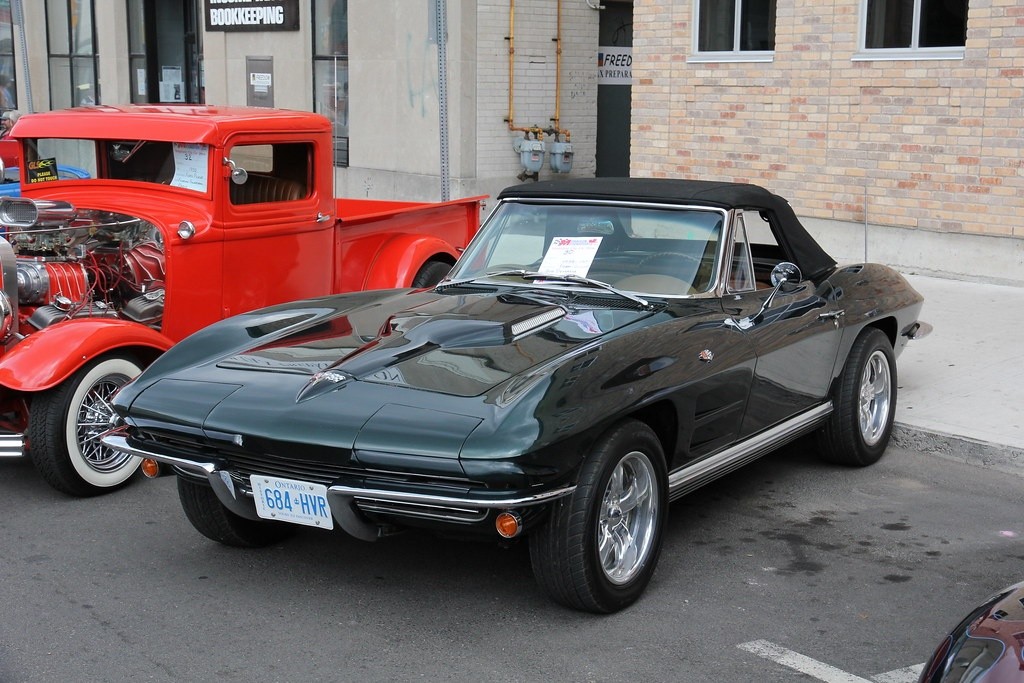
[0,112,17,138]
[0,75,14,108]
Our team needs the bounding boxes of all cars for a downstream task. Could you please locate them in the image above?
[101,176,925,616]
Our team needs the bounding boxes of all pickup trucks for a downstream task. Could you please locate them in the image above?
[0,104,492,500]
